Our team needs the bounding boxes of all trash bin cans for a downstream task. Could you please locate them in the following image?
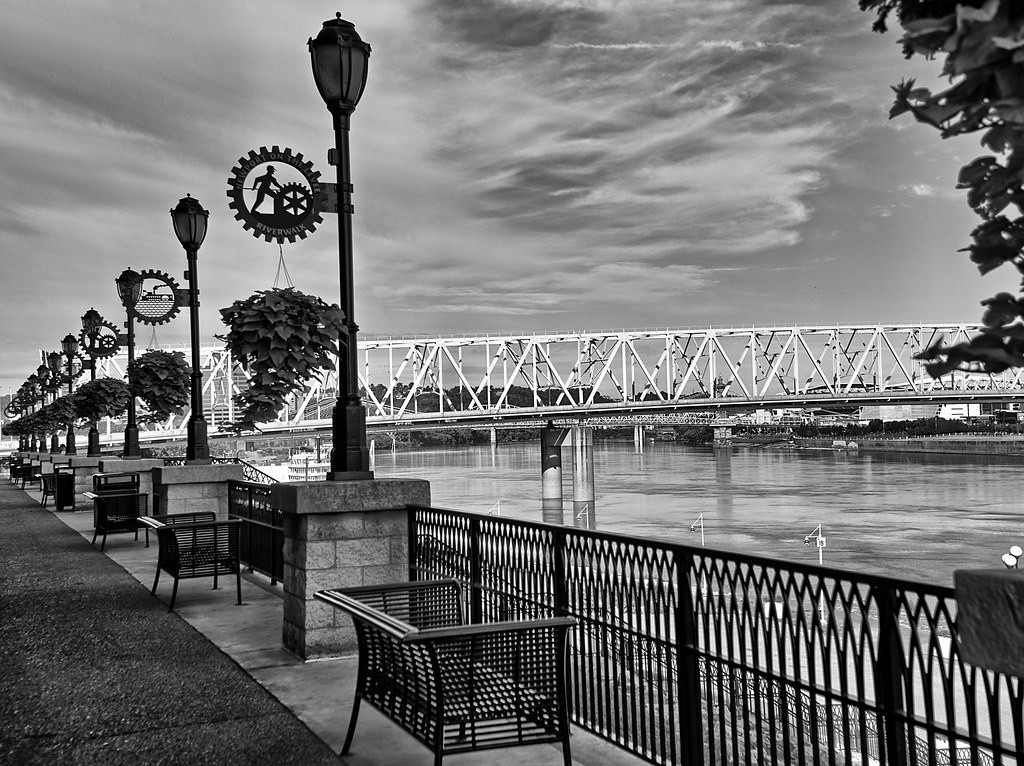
[92,471,140,534]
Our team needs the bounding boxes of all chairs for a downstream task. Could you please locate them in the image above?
[149,511,242,613]
[83,489,150,552]
[314,577,580,766]
[8,457,57,508]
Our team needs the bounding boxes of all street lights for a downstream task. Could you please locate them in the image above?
[16,350,63,455]
[307,9,374,482]
[170,193,215,465]
[60,333,80,456]
[80,306,103,458]
[115,266,144,460]
